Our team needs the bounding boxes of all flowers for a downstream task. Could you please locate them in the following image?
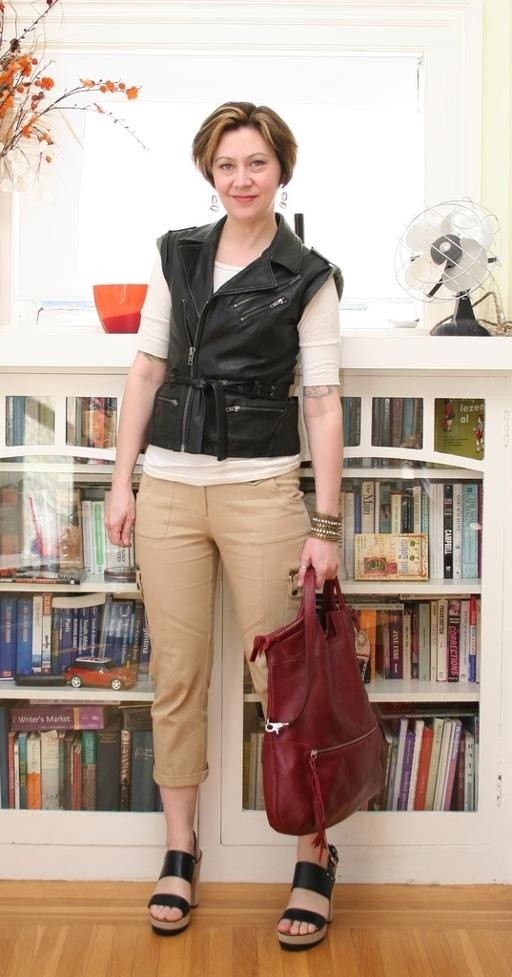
[1,0,147,185]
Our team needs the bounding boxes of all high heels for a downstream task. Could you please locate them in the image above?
[275,842,339,952]
[147,847,203,935]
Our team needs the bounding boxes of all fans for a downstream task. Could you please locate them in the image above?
[398,192,512,337]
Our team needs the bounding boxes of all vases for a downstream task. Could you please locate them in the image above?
[1,186,22,337]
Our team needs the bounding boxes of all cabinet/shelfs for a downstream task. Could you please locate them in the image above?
[0,336,505,880]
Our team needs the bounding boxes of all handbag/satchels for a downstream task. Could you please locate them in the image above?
[249,566,389,836]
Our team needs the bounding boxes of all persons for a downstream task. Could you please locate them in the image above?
[103,101,344,950]
